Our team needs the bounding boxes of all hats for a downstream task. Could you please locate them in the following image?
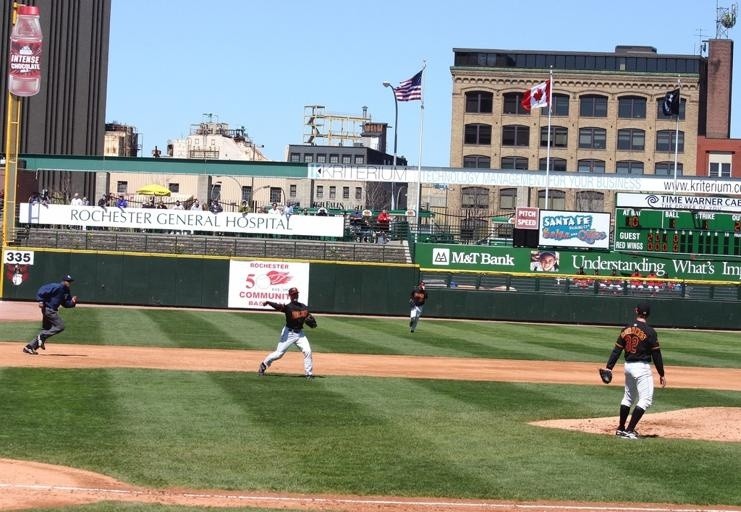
[540,250,556,257]
[634,303,650,317]
[417,282,424,288]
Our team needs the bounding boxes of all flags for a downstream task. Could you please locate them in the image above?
[393,67,424,102]
[519,78,552,110]
[662,87,681,117]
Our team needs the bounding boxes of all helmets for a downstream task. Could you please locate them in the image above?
[289,287,299,295]
[64,273,74,281]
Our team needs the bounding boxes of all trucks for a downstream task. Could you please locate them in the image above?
[475,237,515,247]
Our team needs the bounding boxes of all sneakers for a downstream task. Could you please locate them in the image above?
[38,333,46,349]
[409,321,413,327]
[23,344,38,354]
[621,430,640,439]
[616,429,623,437]
[259,362,266,376]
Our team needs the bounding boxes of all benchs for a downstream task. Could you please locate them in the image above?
[262,205,412,243]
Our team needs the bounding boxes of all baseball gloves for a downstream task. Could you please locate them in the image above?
[305,315,317,328]
[599,368,612,384]
[409,299,415,306]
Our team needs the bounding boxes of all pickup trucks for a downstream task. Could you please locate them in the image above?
[425,233,458,244]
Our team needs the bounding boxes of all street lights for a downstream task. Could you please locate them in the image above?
[383,80,399,211]
[248,142,271,212]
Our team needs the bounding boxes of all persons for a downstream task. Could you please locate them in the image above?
[603,302,667,441]
[142,199,223,237]
[22,274,80,357]
[300,208,327,216]
[98,194,109,231]
[572,263,678,296]
[28,190,50,229]
[258,286,318,379]
[269,202,293,215]
[116,196,127,209]
[407,281,428,333]
[238,200,251,213]
[539,252,558,272]
[350,208,393,231]
[70,193,90,229]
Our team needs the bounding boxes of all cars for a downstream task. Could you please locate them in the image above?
[352,225,384,242]
